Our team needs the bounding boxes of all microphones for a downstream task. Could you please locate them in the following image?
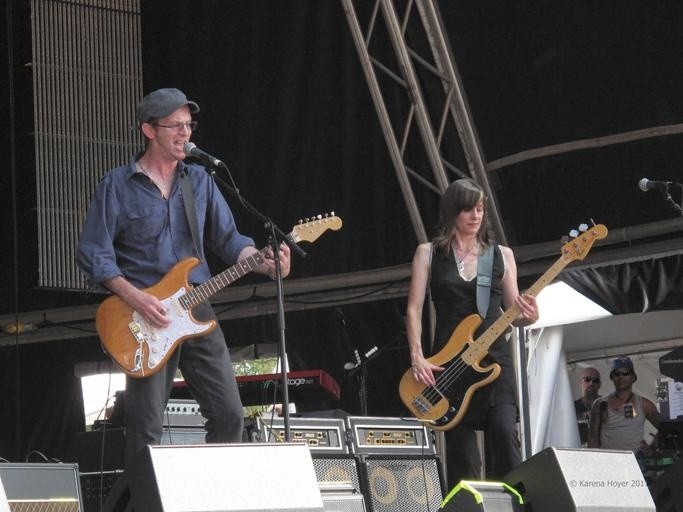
[638,176,673,195]
[181,142,225,168]
[335,306,349,321]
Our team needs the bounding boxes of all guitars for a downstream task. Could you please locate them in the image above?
[398,219,609,431]
[95,212,343,379]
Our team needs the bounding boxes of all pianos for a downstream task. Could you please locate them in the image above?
[170,369,341,404]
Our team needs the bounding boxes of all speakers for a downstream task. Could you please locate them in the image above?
[254,416,363,494]
[345,418,444,512]
[0,462,84,512]
[497,446,657,512]
